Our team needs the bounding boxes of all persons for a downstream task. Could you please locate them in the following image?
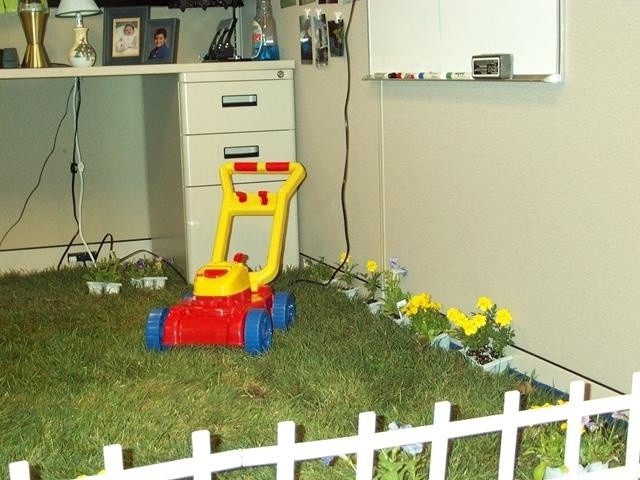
[148,28,169,58]
[114,23,138,52]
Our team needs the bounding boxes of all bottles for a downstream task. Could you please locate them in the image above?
[248,1,280,61]
[373,72,472,80]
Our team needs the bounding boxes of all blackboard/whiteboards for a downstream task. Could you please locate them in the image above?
[361,0,560,83]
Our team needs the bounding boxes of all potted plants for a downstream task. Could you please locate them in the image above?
[85,258,125,294]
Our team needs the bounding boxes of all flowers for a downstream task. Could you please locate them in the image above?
[515,396,630,480]
[398,293,449,334]
[127,256,176,280]
[445,295,516,355]
[335,251,409,306]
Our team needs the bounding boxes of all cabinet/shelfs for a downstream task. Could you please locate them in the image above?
[140,71,301,285]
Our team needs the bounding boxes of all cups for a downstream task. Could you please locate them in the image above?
[16,0,52,67]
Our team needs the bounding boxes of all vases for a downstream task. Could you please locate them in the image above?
[390,315,409,328]
[131,275,169,290]
[430,334,451,352]
[543,463,608,480]
[458,345,513,379]
[364,299,381,314]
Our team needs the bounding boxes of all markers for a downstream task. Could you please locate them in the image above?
[388,72,472,81]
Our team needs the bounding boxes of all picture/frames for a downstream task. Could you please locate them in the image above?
[101,6,181,64]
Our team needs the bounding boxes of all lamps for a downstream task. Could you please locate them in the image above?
[56,0,103,68]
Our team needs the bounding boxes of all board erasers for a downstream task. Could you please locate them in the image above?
[472,54,512,79]
